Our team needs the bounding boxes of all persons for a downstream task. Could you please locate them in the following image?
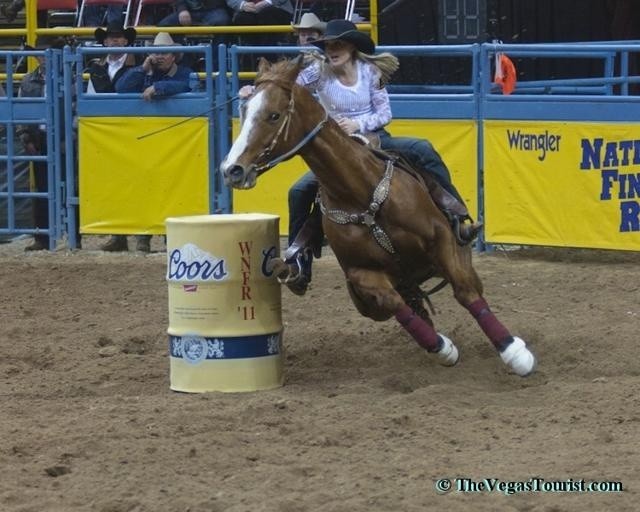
[290,11,330,46]
[79,18,149,253]
[154,1,232,52]
[223,1,296,64]
[76,0,127,27]
[237,18,486,299]
[13,34,79,253]
[113,30,197,253]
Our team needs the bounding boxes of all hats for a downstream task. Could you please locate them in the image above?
[24,35,65,49]
[145,32,184,63]
[95,21,136,46]
[291,13,375,54]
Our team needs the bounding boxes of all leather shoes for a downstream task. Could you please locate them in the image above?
[24,241,48,250]
[270,258,307,296]
[100,240,150,252]
[459,222,483,239]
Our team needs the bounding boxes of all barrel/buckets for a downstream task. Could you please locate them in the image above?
[163,213,286,393]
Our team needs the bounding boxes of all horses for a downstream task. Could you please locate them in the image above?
[219,53,534,378]
